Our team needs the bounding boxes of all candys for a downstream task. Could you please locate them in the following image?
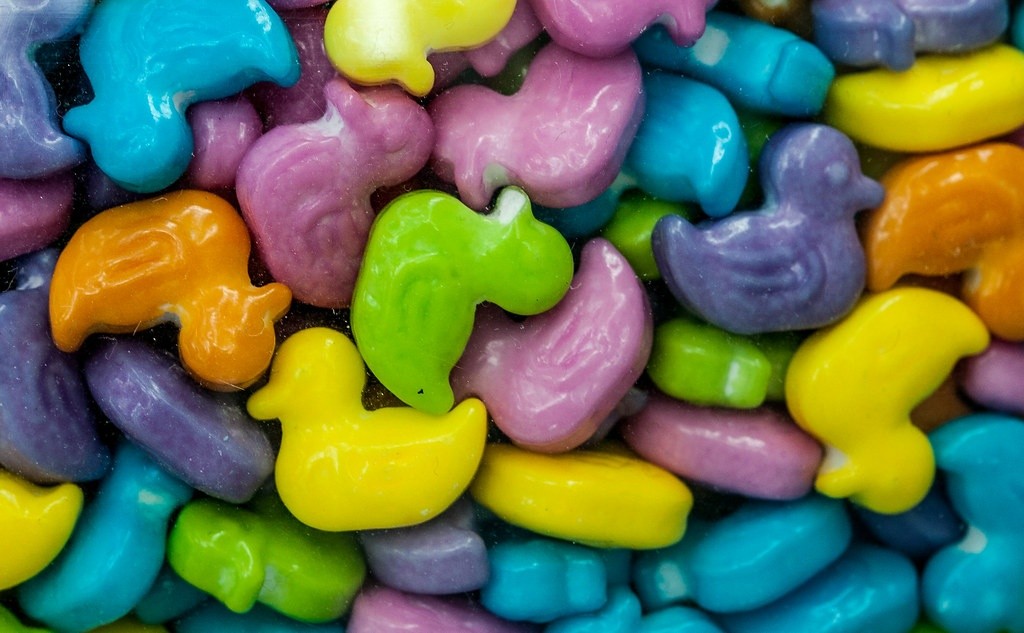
[0,0,1024,633]
